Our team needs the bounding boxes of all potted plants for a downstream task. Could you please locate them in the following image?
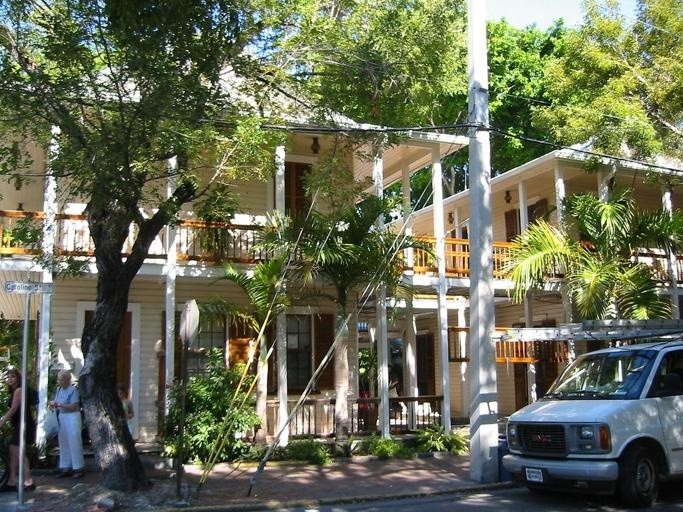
[355,347,393,432]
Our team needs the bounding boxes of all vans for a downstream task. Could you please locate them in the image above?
[501,339,681,509]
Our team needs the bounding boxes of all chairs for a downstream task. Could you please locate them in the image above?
[654,373,682,396]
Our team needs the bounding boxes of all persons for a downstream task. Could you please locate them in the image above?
[117,382,134,435]
[0,367,37,491]
[47,368,85,479]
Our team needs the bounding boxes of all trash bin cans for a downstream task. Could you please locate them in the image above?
[61,202,93,254]
[499,436,512,482]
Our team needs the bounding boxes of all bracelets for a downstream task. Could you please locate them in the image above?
[1,416,8,422]
[58,403,61,407]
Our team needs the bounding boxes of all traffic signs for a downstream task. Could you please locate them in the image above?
[4,281,55,293]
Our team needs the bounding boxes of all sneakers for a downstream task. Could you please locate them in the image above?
[55,468,84,481]
[1,483,37,493]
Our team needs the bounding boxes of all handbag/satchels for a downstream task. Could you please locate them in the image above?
[44,403,59,439]
